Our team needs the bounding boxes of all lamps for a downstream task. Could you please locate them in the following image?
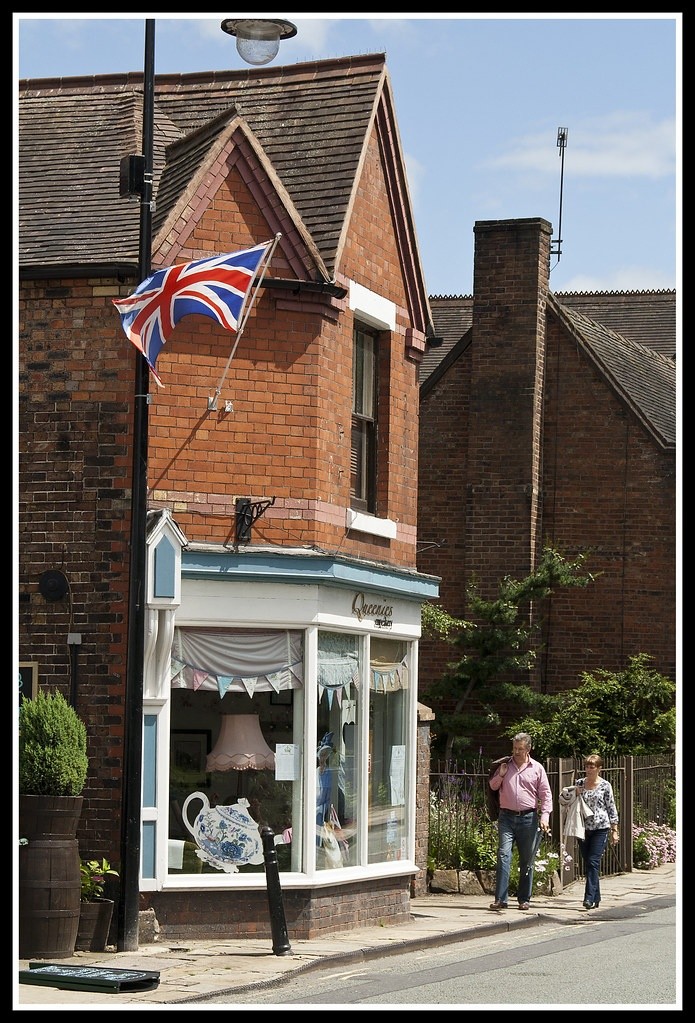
[207,713,275,803]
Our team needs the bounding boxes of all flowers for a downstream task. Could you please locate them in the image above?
[77,857,120,903]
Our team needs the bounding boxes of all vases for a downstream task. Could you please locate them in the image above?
[74,896,115,951]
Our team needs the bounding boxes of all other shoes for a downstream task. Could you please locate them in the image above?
[583,899,599,909]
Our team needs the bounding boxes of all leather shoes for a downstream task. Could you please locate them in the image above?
[518,902,529,910]
[489,899,508,909]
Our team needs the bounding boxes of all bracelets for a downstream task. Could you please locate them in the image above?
[612,828,618,832]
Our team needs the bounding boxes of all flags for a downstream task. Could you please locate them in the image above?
[112,238,274,389]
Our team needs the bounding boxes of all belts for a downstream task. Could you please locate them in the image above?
[500,808,538,817]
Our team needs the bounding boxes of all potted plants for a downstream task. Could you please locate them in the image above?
[19,686,90,839]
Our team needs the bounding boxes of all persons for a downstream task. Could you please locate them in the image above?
[169,779,187,830]
[562,754,619,909]
[315,747,333,846]
[210,793,268,836]
[489,733,553,910]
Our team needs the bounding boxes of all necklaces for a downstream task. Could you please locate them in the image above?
[319,763,326,774]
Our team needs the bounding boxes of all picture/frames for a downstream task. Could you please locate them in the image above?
[170,729,213,787]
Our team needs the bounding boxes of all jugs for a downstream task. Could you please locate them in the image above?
[182,791,285,872]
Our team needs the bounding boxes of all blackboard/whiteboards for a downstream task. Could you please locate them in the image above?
[28,962,161,995]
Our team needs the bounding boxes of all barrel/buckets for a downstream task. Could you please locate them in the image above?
[19,839,81,959]
